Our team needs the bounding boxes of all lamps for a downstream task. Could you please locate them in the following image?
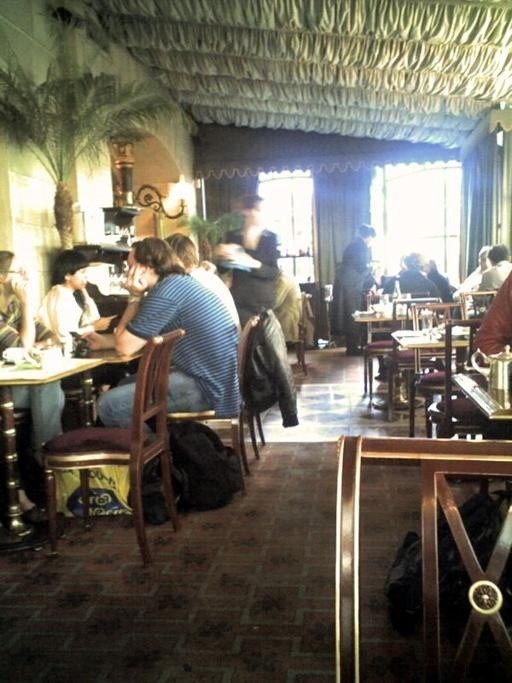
[132,174,197,221]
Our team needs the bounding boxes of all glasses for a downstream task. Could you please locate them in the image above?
[1,268,26,276]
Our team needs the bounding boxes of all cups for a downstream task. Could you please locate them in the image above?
[3,347,24,364]
[41,350,63,374]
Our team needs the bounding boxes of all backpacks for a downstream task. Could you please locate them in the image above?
[383,487,509,638]
[167,420,246,513]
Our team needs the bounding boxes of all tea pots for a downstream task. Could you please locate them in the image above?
[472,346,512,411]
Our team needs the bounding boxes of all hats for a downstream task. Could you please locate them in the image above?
[214,242,262,272]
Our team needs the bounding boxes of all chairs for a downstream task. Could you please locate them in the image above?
[46,327,188,567]
[459,291,499,320]
[285,291,312,377]
[164,313,262,495]
[411,301,476,436]
[364,296,442,399]
[334,434,512,683]
[428,320,489,441]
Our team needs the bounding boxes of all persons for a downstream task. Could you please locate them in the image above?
[422,258,450,302]
[214,193,280,325]
[466,243,512,318]
[0,248,66,523]
[165,232,242,334]
[472,271,512,364]
[382,252,441,297]
[79,236,243,433]
[37,249,118,344]
[273,267,303,342]
[329,224,377,356]
[451,245,492,318]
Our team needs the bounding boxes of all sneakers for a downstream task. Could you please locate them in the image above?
[26,504,63,524]
[347,345,365,355]
